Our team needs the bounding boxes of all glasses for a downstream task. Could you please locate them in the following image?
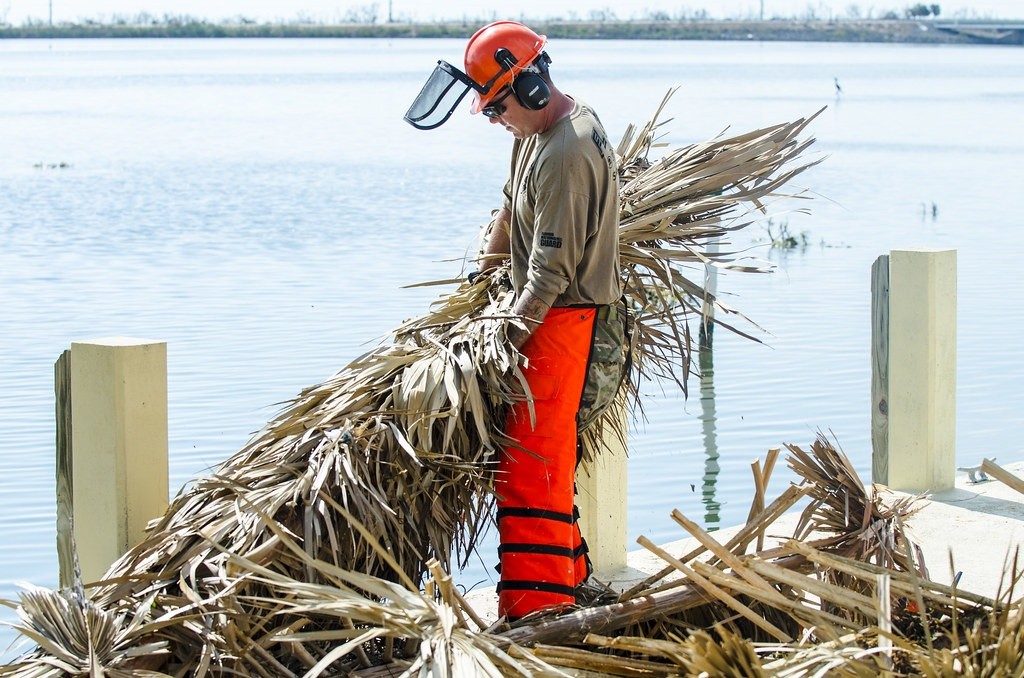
[482,88,513,118]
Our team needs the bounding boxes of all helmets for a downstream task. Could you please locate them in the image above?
[464,20,547,116]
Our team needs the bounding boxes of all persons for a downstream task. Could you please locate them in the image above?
[464,20,623,634]
